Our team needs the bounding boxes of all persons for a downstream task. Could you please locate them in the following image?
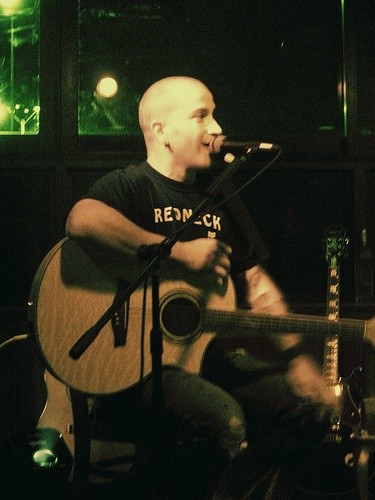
[65,76,339,500]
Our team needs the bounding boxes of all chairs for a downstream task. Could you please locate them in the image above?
[67,382,188,500]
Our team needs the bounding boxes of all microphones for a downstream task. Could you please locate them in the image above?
[209,135,280,155]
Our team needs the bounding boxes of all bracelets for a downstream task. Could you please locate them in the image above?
[280,342,311,364]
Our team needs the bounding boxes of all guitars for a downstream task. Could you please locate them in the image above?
[321,236,349,429]
[27,234,375,395]
[36,369,94,460]
